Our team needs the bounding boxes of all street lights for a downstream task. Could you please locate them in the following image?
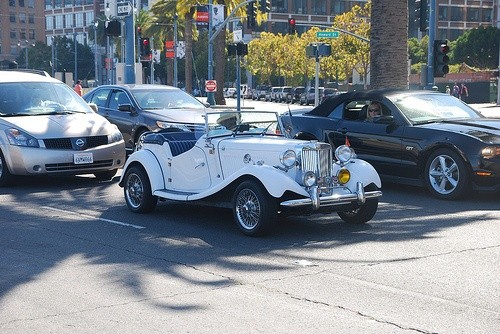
[17,39,36,68]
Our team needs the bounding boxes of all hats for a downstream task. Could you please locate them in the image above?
[216,110,236,124]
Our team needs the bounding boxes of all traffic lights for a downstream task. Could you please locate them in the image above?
[104,21,121,36]
[288,18,296,36]
[413,0,427,31]
[141,38,149,55]
[305,44,332,58]
[245,5,257,29]
[435,40,450,77]
[226,42,248,57]
[259,0,270,14]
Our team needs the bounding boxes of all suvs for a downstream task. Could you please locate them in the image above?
[0,68,125,187]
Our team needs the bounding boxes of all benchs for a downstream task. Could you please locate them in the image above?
[95,97,118,108]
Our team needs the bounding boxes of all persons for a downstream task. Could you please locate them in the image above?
[451,82,460,98]
[217,109,238,129]
[459,83,468,100]
[362,101,383,123]
[74,80,83,96]
[446,86,451,94]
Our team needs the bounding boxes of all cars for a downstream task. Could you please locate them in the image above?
[81,83,220,152]
[223,84,339,105]
[276,89,500,201]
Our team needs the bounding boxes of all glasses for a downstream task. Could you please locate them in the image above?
[368,109,378,112]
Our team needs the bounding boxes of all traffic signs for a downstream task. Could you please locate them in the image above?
[116,2,133,17]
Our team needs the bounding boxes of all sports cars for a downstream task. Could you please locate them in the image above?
[109,111,383,235]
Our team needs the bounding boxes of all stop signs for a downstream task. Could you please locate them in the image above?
[206,81,216,92]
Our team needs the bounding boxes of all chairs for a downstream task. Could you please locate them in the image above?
[355,105,368,122]
[118,93,131,104]
[0,87,19,114]
[167,140,198,157]
